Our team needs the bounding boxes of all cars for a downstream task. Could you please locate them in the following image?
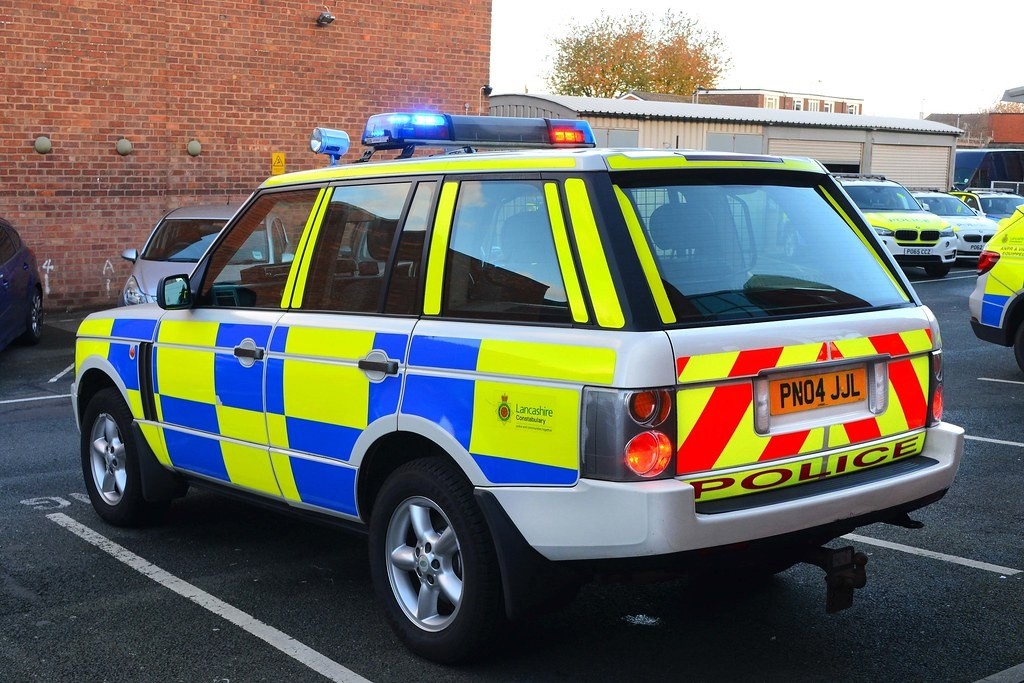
[120,204,296,308]
[0,217,44,352]
[906,187,998,261]
[945,188,1024,224]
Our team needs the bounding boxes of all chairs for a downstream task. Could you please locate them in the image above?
[346,215,424,312]
[649,202,730,300]
[501,211,568,303]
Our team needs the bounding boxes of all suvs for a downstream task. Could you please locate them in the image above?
[969,202,1024,373]
[784,173,957,278]
[78,105,966,661]
